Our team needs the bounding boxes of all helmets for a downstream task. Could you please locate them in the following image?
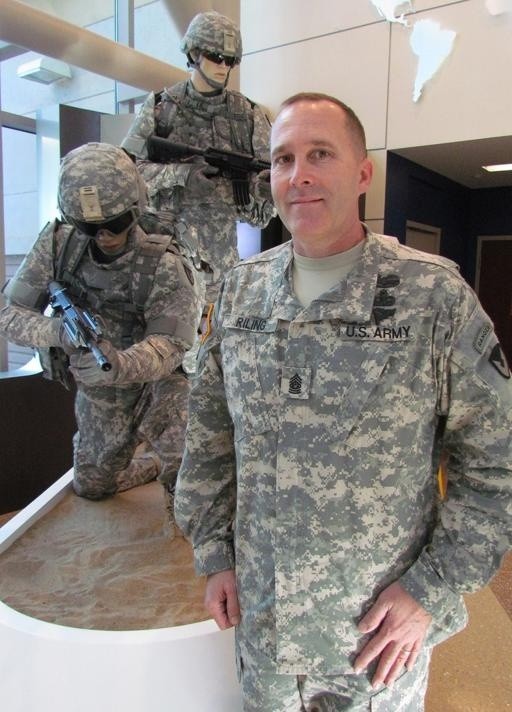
[181,10,243,65]
[56,142,148,224]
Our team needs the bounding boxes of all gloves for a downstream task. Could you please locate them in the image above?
[68,338,118,388]
[61,314,107,357]
[183,164,220,196]
[254,169,273,203]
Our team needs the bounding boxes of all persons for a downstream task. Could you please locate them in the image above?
[0,143,206,500]
[171,93,510,710]
[115,15,279,384]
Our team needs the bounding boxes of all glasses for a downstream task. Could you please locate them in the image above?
[200,52,236,67]
[65,207,139,240]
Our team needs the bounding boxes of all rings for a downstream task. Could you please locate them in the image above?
[401,649,410,655]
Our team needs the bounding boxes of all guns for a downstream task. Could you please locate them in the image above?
[147,134,270,205]
[48,280,111,372]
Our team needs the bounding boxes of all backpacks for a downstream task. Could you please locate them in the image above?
[51,210,207,329]
